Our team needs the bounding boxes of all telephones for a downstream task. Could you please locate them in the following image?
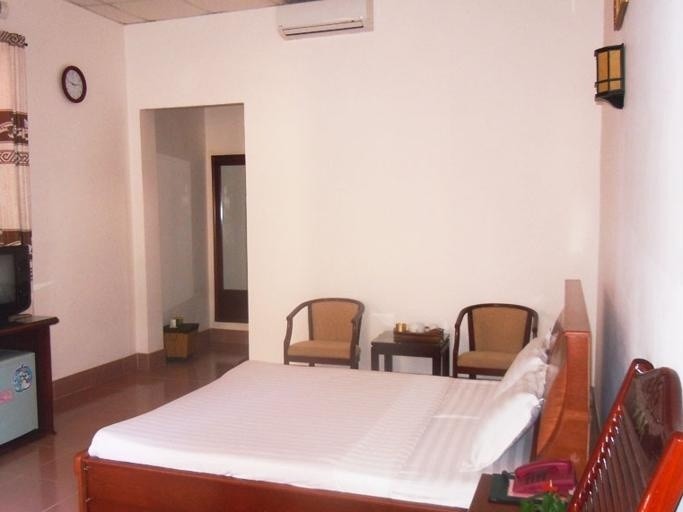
[512,460,574,495]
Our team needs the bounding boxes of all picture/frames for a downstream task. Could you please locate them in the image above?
[614,0,629,30]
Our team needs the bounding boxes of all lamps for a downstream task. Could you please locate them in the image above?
[594,43,624,109]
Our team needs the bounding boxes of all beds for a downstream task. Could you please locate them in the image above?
[75,278,591,512]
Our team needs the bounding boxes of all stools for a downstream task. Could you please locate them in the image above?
[163,324,199,360]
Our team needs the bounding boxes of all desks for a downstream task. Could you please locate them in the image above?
[371,330,450,376]
[0,315,59,457]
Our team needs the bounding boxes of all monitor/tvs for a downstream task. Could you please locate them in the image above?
[0,246,31,326]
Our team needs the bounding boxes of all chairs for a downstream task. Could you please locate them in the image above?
[283,297,364,369]
[453,303,539,377]
[568,359,683,512]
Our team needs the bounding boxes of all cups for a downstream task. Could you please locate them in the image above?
[170,314,184,329]
[397,323,407,334]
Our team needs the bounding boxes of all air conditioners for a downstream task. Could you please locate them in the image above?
[276,0,373,40]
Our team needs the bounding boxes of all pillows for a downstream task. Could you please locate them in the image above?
[463,329,550,471]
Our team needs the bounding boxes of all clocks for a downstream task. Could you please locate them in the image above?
[62,65,86,103]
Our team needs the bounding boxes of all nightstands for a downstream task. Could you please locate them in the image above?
[469,474,576,512]
